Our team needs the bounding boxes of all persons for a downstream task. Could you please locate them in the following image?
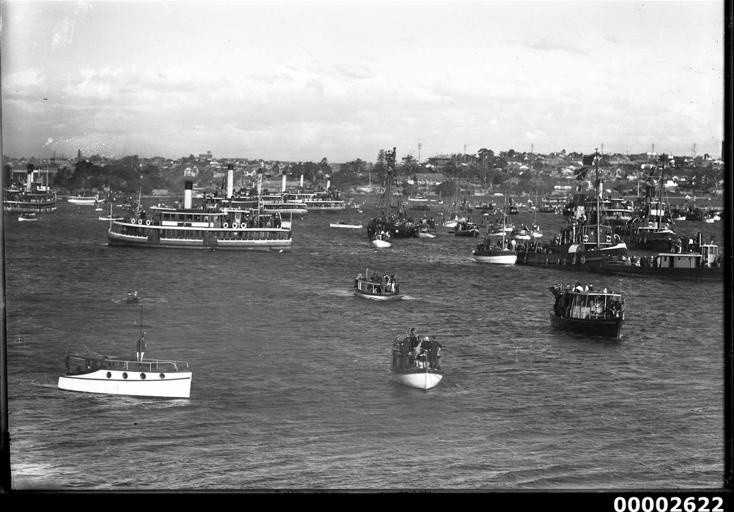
[391,325,444,371]
[368,270,397,294]
[549,281,625,320]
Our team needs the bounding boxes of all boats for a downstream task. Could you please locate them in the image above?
[391,334,443,390]
[442,216,479,236]
[3,164,57,213]
[354,268,403,301]
[372,234,392,248]
[67,197,96,206]
[18,213,38,222]
[367,147,437,238]
[330,224,363,229]
[472,148,723,278]
[57,305,192,399]
[108,165,346,253]
[99,216,124,221]
[549,286,623,337]
